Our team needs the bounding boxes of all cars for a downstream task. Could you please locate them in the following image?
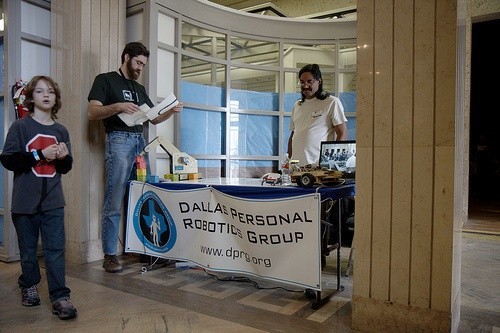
[291,164,343,187]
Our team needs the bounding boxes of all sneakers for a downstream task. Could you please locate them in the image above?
[52,298,78,320]
[21,285,41,305]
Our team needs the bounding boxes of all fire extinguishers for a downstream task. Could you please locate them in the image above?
[11,80,31,120]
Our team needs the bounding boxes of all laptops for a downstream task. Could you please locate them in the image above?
[318,140,356,184]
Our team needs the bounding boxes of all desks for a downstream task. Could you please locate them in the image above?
[127,178,356,310]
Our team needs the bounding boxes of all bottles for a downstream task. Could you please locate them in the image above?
[281,154,292,186]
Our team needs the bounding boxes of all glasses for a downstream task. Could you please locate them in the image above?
[297,79,316,86]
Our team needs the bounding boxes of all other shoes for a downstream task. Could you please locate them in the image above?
[103,257,123,272]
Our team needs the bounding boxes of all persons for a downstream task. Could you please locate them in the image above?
[87,42,183,272]
[287,64,348,170]
[1,75,79,322]
